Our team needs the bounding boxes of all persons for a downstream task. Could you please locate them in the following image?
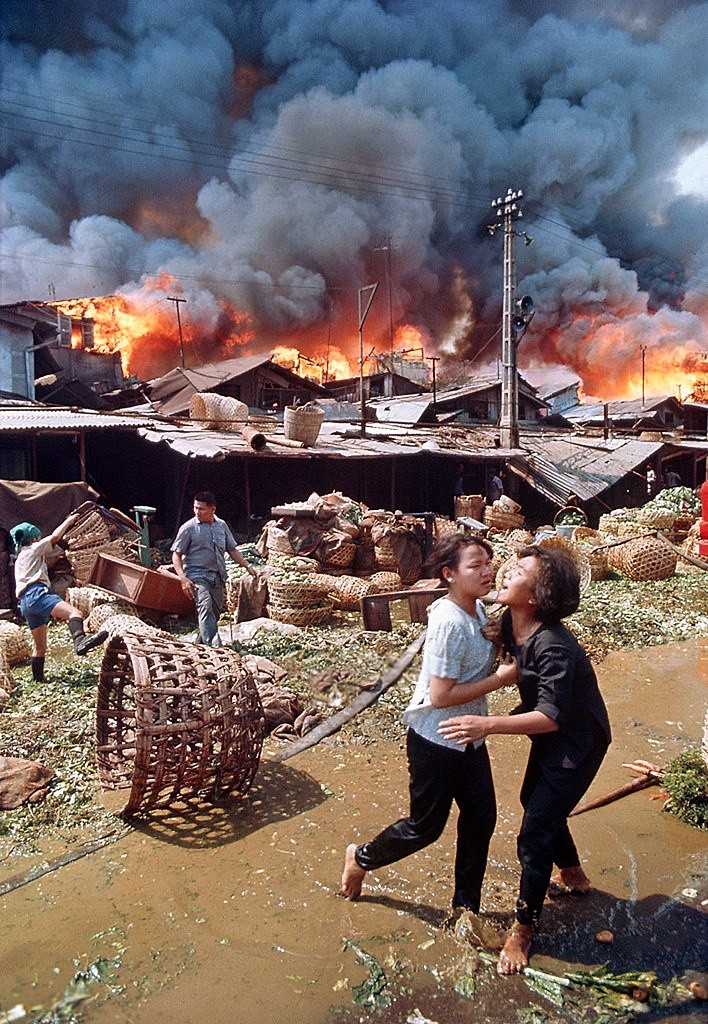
[10,513,109,677]
[647,463,681,495]
[488,468,503,506]
[169,492,258,649]
[342,536,517,916]
[434,538,611,972]
[447,464,464,504]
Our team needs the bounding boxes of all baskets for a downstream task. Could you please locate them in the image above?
[332,575,378,611]
[325,542,356,566]
[375,534,407,565]
[607,536,677,580]
[454,496,485,521]
[266,598,335,625]
[483,505,525,533]
[371,571,402,600]
[93,631,262,817]
[67,587,117,618]
[265,525,323,556]
[267,577,335,608]
[189,393,220,429]
[571,526,606,564]
[284,402,325,447]
[216,396,249,432]
[64,511,127,584]
[637,511,675,529]
[248,415,279,433]
[98,615,149,652]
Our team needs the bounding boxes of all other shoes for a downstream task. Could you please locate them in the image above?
[73,630,109,656]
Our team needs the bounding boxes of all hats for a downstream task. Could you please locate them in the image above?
[10,522,41,552]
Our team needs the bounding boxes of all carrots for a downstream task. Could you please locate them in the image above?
[648,792,668,802]
[621,759,663,775]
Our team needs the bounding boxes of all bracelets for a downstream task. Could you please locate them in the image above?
[247,563,252,570]
